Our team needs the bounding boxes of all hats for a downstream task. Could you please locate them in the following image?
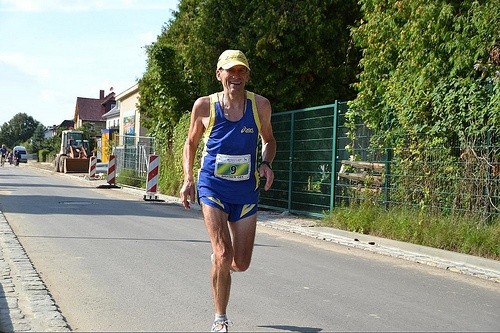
[217,49,250,71]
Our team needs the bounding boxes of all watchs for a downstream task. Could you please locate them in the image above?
[259,161,273,170]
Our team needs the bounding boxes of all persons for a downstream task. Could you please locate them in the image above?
[180,50,276,332]
[0,144,21,167]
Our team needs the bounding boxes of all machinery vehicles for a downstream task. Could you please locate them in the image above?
[54,129,90,174]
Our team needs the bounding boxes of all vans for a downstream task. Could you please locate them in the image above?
[13,146,27,163]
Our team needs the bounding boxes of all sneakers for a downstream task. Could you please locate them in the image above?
[211,312,233,333]
